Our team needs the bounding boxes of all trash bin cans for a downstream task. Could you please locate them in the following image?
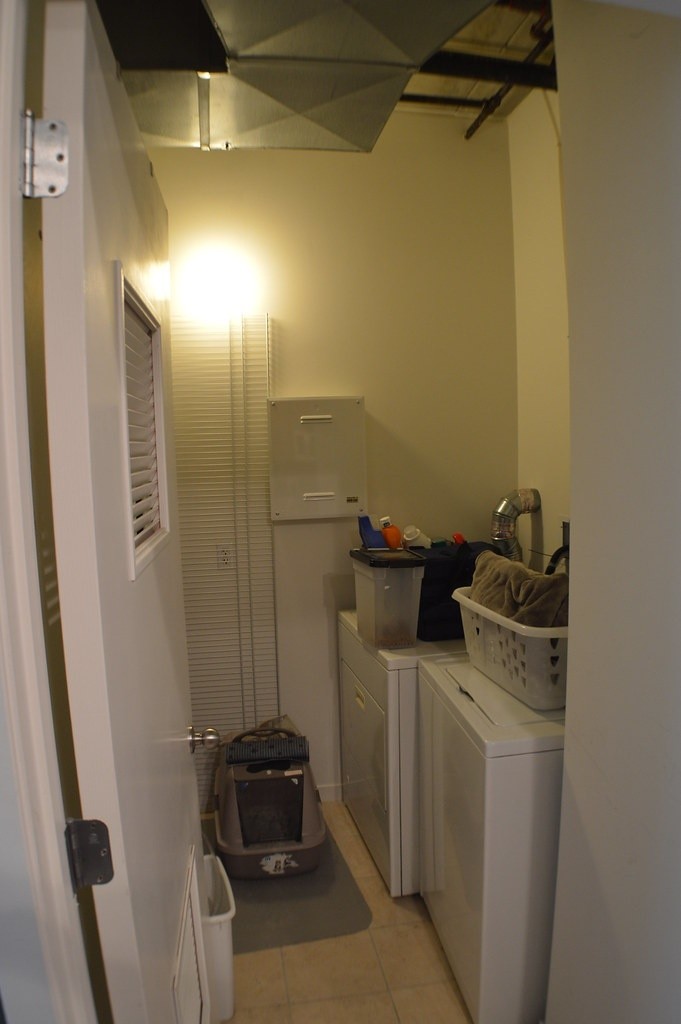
[199,853,237,1024]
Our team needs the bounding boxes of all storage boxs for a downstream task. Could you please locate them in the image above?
[348,546,428,649]
[201,852,236,1023]
[418,540,505,643]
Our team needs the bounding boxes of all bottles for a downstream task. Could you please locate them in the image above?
[379,516,401,548]
[403,524,432,548]
